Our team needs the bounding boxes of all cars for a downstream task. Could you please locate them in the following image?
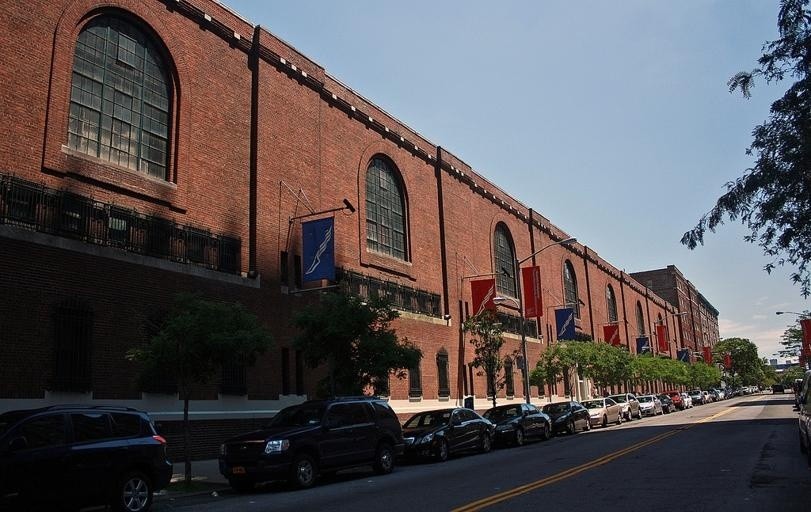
[774,383,785,394]
[580,397,623,428]
[542,401,592,436]
[481,403,553,448]
[1,403,173,511]
[607,393,643,421]
[636,383,759,415]
[394,407,496,464]
[791,370,811,468]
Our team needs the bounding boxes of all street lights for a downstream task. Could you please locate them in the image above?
[516,237,577,404]
[654,310,688,391]
[777,311,811,317]
[702,337,724,389]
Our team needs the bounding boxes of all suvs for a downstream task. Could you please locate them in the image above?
[219,395,404,495]
